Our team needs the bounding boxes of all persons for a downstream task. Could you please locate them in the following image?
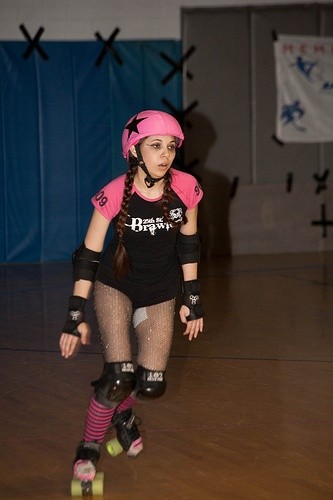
[68,109,206,483]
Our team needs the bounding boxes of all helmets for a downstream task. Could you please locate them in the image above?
[122,110,184,157]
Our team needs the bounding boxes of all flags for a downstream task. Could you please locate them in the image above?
[270,35,333,145]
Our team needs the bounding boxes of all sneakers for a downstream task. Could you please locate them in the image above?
[113,408,143,456]
[72,441,101,480]
